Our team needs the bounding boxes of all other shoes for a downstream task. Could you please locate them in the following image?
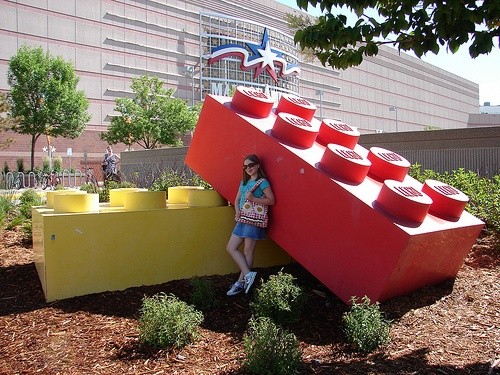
[244,272,257,294]
[226,280,244,295]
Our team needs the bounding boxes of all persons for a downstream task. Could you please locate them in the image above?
[101,146,122,176]
[226,154,275,295]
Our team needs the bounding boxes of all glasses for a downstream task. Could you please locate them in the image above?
[243,163,257,169]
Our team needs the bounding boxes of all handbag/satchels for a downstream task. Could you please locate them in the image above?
[235,178,276,228]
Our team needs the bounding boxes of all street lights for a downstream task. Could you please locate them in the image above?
[314,89,324,122]
[388,104,399,133]
